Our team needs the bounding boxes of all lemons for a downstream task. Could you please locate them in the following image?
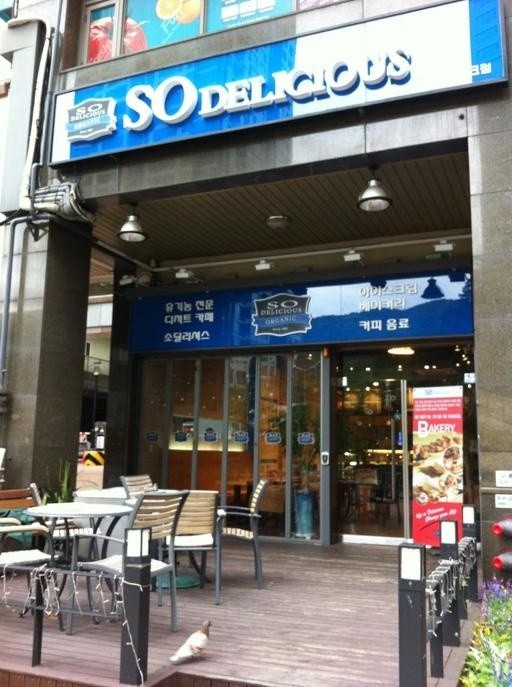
[155,0,200,24]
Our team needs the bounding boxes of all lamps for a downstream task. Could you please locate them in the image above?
[116,199,148,243]
[354,162,392,213]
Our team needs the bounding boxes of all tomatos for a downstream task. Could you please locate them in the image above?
[89,16,146,62]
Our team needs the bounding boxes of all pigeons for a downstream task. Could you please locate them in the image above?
[168,618,212,664]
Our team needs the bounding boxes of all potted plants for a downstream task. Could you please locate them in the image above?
[266,395,320,538]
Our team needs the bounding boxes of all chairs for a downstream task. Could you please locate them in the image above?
[155,489,227,608]
[0,473,188,634]
[197,476,268,587]
